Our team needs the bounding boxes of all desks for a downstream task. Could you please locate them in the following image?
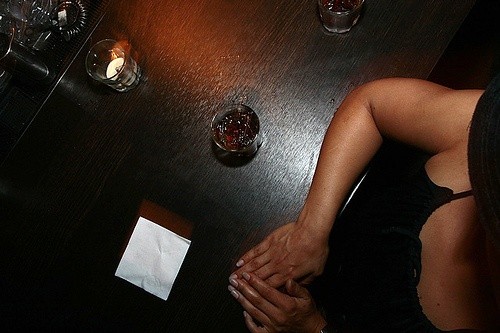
[0,0,478,333]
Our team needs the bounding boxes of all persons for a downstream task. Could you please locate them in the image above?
[228,78,500,333]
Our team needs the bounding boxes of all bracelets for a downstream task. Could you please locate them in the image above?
[320,324,337,333]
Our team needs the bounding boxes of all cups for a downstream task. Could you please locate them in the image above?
[7,0,55,26]
[317,0,365,34]
[211,104,264,158]
[85,39,140,93]
